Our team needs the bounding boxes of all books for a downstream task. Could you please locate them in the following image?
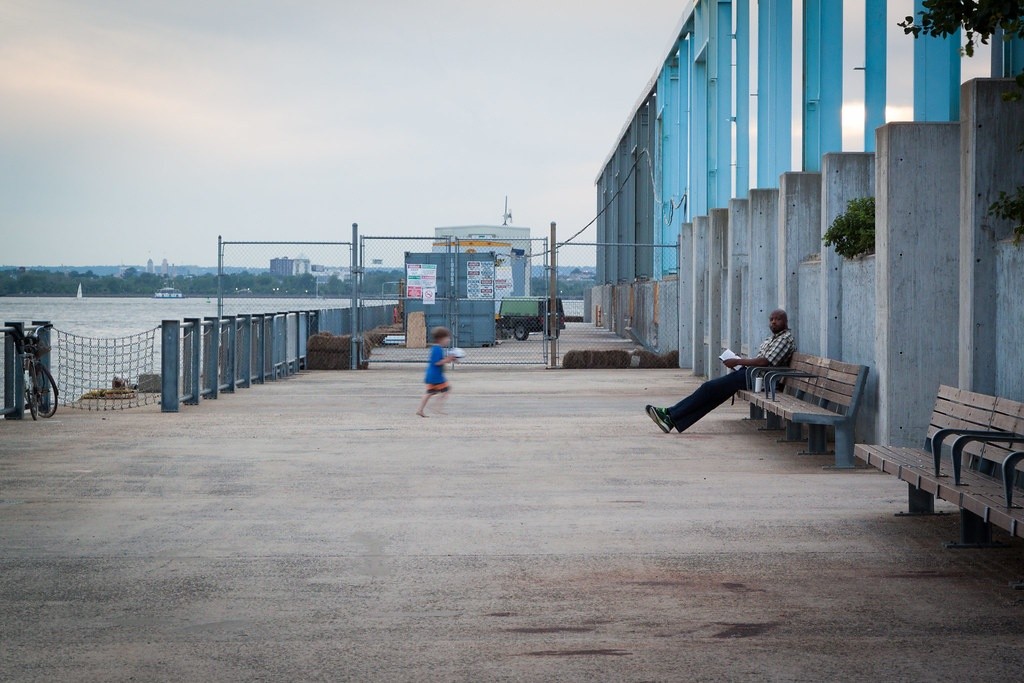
[718,349,743,371]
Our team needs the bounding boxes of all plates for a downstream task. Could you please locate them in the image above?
[450,355,466,358]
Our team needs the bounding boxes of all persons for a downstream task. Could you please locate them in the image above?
[646,309,794,432]
[415,326,458,418]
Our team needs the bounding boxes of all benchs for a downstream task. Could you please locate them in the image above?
[854,385,1024,549]
[738,352,870,470]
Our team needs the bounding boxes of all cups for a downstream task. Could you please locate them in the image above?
[755,378,762,393]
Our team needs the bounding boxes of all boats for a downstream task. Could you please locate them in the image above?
[154,287,182,298]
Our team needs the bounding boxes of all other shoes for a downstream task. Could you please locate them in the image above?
[646,405,668,415]
[649,407,674,433]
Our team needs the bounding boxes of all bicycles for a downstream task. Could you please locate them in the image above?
[12,323,59,421]
